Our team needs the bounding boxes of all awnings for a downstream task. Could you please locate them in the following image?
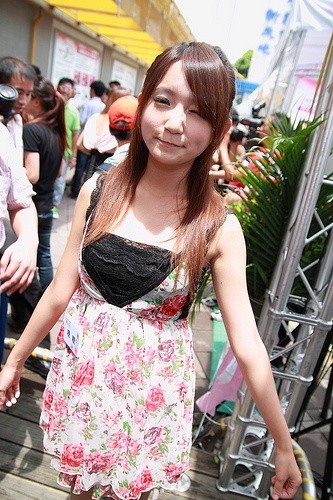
[44,0,163,65]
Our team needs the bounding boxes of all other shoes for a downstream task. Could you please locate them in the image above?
[7,313,26,330]
[24,357,52,376]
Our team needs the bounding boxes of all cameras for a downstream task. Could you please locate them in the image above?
[0,83,18,125]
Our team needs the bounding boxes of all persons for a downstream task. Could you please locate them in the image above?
[109,81,120,89]
[201,108,290,320]
[52,78,81,218]
[68,81,107,199]
[22,75,67,295]
[0,57,51,376]
[0,42,302,500]
[76,85,130,176]
[93,95,140,176]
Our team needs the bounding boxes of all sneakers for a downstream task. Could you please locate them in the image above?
[51,206,59,218]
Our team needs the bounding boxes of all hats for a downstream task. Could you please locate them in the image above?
[108,96,138,131]
